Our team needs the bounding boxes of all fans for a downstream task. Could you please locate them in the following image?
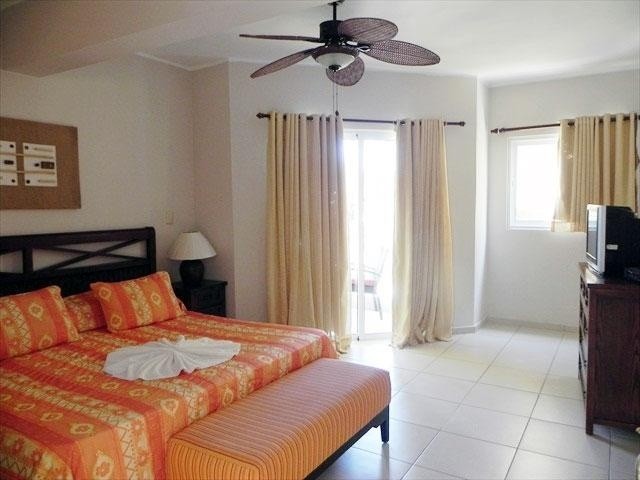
[239,1,441,89]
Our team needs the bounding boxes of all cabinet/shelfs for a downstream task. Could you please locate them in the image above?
[577,260,640,436]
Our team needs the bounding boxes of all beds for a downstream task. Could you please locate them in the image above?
[1,226,329,480]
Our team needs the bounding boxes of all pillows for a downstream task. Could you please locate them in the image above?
[90,269,181,332]
[1,286,81,362]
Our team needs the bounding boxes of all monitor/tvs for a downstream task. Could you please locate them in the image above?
[585,204,640,279]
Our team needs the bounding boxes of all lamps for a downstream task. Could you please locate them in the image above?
[166,230,217,287]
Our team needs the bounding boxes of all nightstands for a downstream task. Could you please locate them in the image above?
[166,279,229,318]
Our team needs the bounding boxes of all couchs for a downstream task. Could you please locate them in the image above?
[169,356,391,479]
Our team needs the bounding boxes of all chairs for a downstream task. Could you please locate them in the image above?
[352,267,383,320]
